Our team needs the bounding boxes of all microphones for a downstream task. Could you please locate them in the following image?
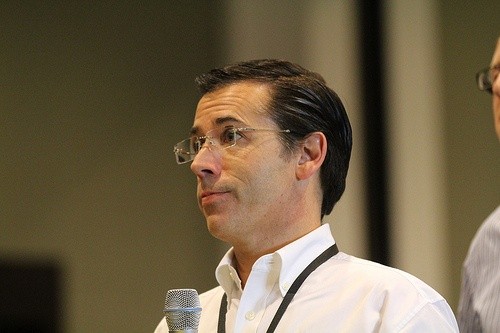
[163,289,202,333]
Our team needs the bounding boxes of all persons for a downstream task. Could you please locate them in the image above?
[458,36,500,333]
[153,58,460,332]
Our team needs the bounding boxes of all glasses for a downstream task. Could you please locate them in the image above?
[172,125,306,164]
[476,62,500,95]
